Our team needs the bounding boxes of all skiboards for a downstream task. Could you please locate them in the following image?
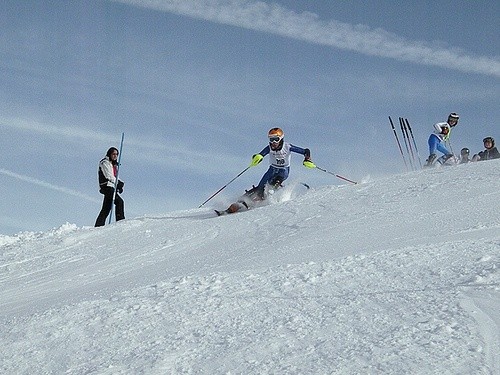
[213,199,252,216]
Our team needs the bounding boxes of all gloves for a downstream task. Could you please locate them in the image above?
[303,159,313,166]
[440,127,449,135]
[253,155,262,162]
[116,180,124,194]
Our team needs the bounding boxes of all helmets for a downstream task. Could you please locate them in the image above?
[447,113,459,127]
[461,148,470,155]
[482,137,494,149]
[269,128,284,140]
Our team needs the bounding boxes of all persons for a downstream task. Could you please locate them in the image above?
[219,127,314,215]
[94,147,126,228]
[423,112,500,166]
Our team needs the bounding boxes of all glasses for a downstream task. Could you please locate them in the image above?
[449,117,457,122]
[270,137,281,143]
